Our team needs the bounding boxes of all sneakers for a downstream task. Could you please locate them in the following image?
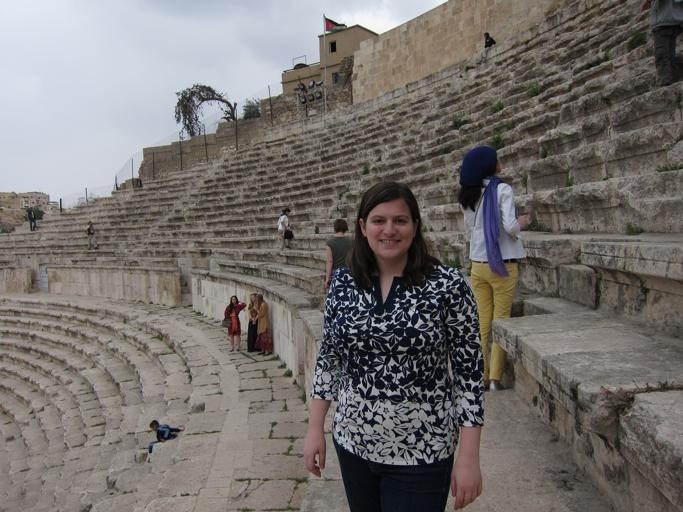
[490,384,503,390]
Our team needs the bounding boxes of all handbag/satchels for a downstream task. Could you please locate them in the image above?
[284,230,293,239]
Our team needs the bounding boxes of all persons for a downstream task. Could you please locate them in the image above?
[277,207,292,251]
[86,221,98,250]
[26,207,36,231]
[248,292,261,352]
[324,218,354,289]
[639,0,683,86]
[253,293,272,355]
[483,32,497,48]
[223,295,247,352]
[148,419,185,453]
[459,144,532,392]
[302,181,485,511]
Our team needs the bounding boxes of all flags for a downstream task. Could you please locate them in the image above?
[325,16,347,32]
